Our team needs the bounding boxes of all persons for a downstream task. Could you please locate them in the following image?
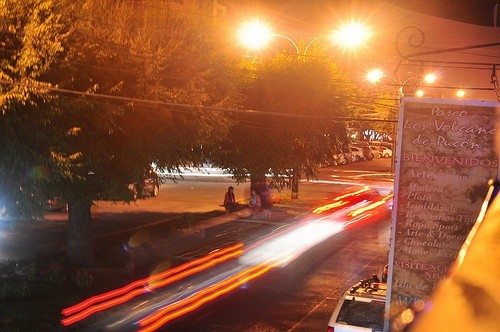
[378,143,385,158]
[381,264,388,283]
[363,144,371,161]
[248,189,262,220]
[375,144,380,158]
[223,186,238,215]
[260,189,273,220]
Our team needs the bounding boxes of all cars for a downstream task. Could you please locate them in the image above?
[45,195,69,214]
[332,139,392,162]
[100,164,159,198]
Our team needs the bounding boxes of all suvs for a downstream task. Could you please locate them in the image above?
[327,275,386,332]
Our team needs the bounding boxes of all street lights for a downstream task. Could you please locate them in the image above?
[414,89,468,100]
[366,67,439,101]
[240,19,369,199]
